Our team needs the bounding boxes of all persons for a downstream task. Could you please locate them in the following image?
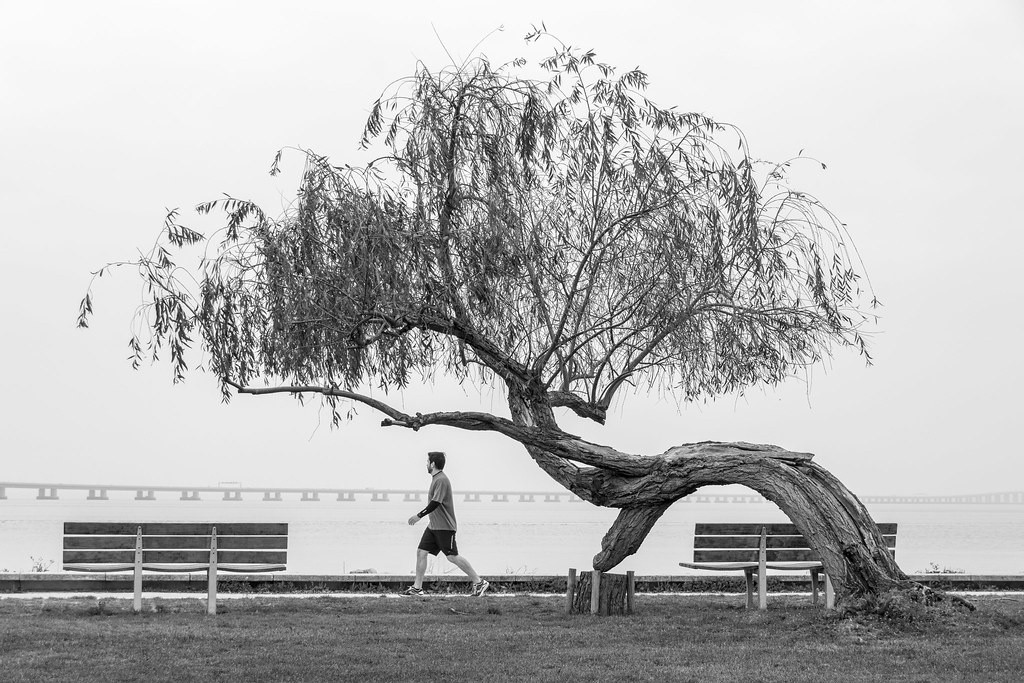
[398,451,490,599]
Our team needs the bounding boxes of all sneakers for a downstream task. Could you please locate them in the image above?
[399,587,424,597]
[471,579,489,596]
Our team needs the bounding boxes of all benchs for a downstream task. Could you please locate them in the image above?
[64,521,288,614]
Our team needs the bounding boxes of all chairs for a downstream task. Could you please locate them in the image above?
[679,523,897,610]
